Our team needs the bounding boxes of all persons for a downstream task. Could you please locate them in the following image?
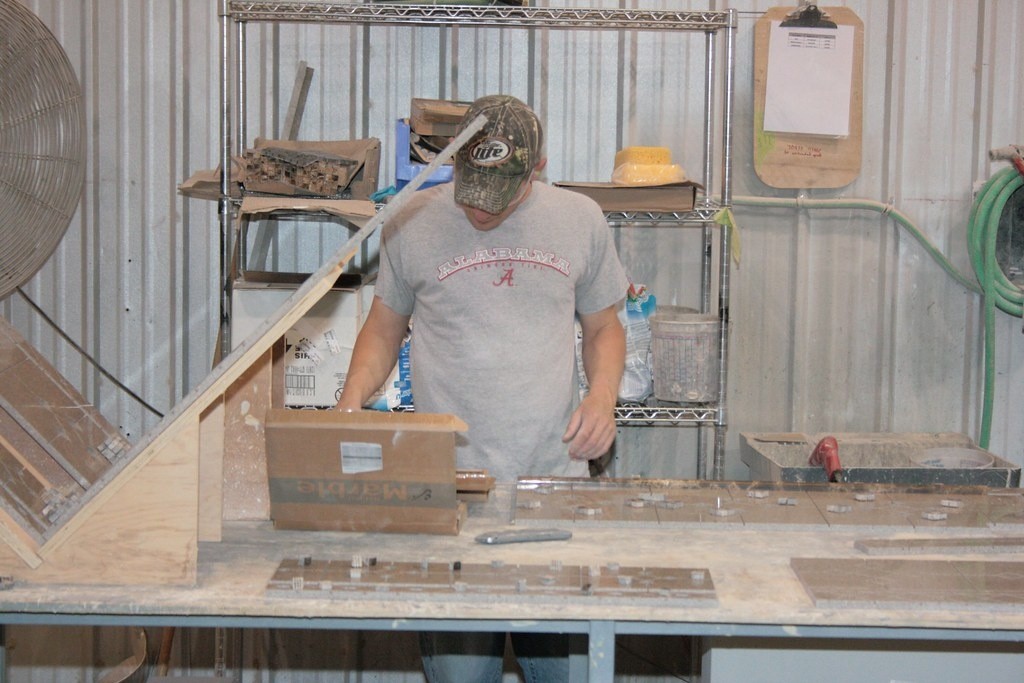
[335,94,635,683]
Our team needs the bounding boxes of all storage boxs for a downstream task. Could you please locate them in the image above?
[228,211,379,406]
[410,98,473,136]
[552,180,706,214]
[264,407,469,537]
[243,137,382,201]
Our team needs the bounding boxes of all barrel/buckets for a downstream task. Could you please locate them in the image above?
[650,314,722,403]
[910,446,995,468]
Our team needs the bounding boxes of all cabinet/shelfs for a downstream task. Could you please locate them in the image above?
[216,0,738,478]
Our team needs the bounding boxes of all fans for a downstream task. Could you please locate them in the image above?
[0,0,90,302]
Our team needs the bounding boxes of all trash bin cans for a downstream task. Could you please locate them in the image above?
[650,315,733,404]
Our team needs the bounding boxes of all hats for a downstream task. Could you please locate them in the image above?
[453,94,543,215]
